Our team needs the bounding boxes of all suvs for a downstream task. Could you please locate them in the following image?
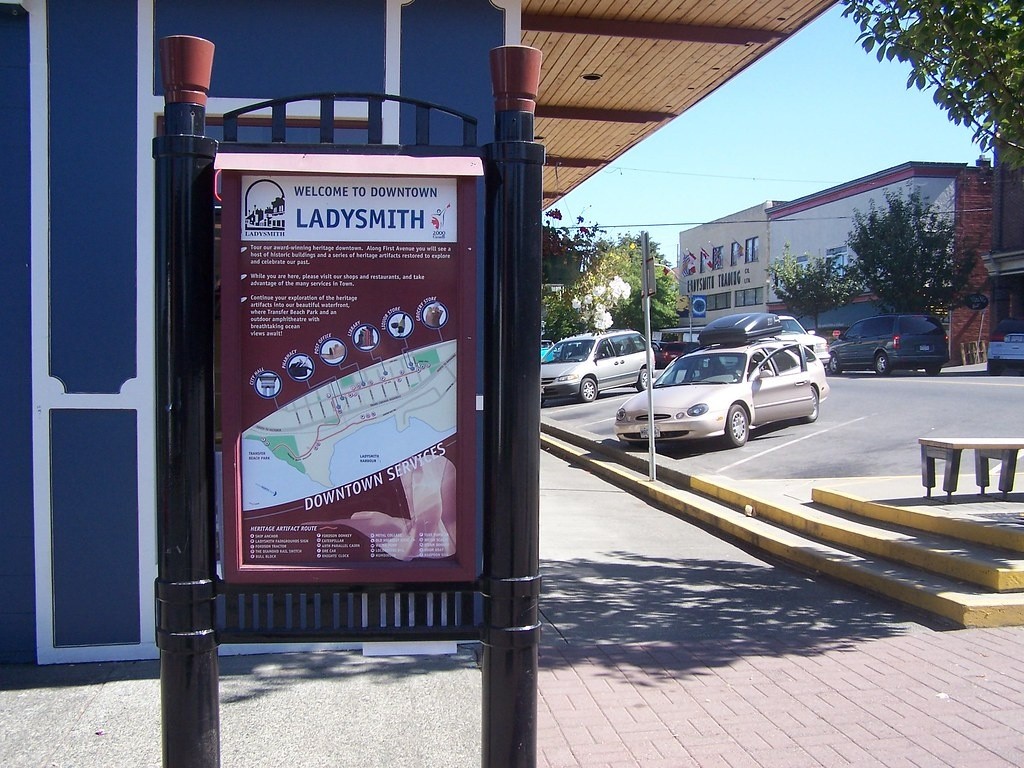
[758,316,831,367]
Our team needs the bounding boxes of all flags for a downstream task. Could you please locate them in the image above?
[700,247,723,270]
[663,267,670,276]
[681,252,697,278]
[734,242,744,257]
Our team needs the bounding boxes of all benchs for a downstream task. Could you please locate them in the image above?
[918,438,1024,505]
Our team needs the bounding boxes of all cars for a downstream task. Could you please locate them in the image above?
[614,336,831,447]
[662,342,700,367]
[986,317,1024,376]
[541,340,557,362]
[651,341,666,369]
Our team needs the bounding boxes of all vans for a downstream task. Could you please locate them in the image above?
[541,330,656,406]
[828,313,950,377]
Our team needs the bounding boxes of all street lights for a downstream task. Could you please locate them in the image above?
[653,264,693,342]
